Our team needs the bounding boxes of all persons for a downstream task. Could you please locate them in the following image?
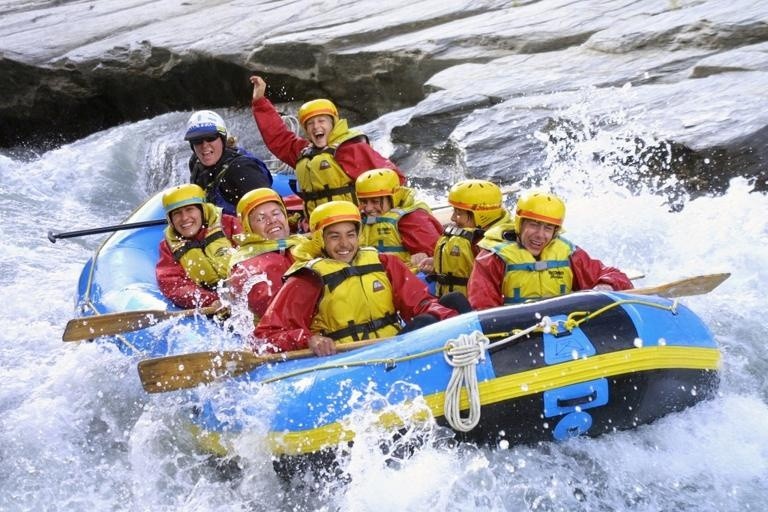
[249,74,407,232]
[227,188,314,321]
[254,199,471,360]
[153,184,244,313]
[355,168,445,273]
[415,179,516,301]
[466,190,633,311]
[185,109,273,213]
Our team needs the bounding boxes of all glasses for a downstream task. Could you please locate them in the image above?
[191,136,218,145]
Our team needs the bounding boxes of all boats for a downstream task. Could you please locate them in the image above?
[77,171,722,487]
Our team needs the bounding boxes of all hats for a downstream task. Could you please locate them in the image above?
[514,191,565,234]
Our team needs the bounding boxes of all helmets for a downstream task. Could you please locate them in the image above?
[236,188,288,233]
[184,110,227,141]
[309,201,362,232]
[355,168,402,208]
[299,99,339,125]
[162,184,209,227]
[448,181,502,228]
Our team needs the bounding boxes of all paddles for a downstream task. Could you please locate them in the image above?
[138,336,397,393]
[612,273,732,297]
[47,194,305,243]
[62,307,224,341]
